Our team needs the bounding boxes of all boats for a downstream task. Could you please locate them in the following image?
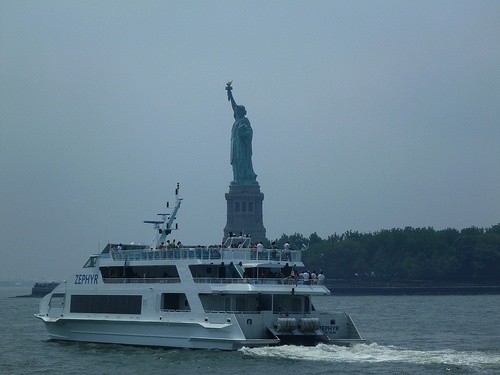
[33,183,378,351]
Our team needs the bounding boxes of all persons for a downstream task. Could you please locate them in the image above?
[270,271,284,279]
[291,264,325,285]
[257,242,263,252]
[284,242,291,262]
[111,244,122,250]
[227,91,256,178]
[159,239,182,249]
